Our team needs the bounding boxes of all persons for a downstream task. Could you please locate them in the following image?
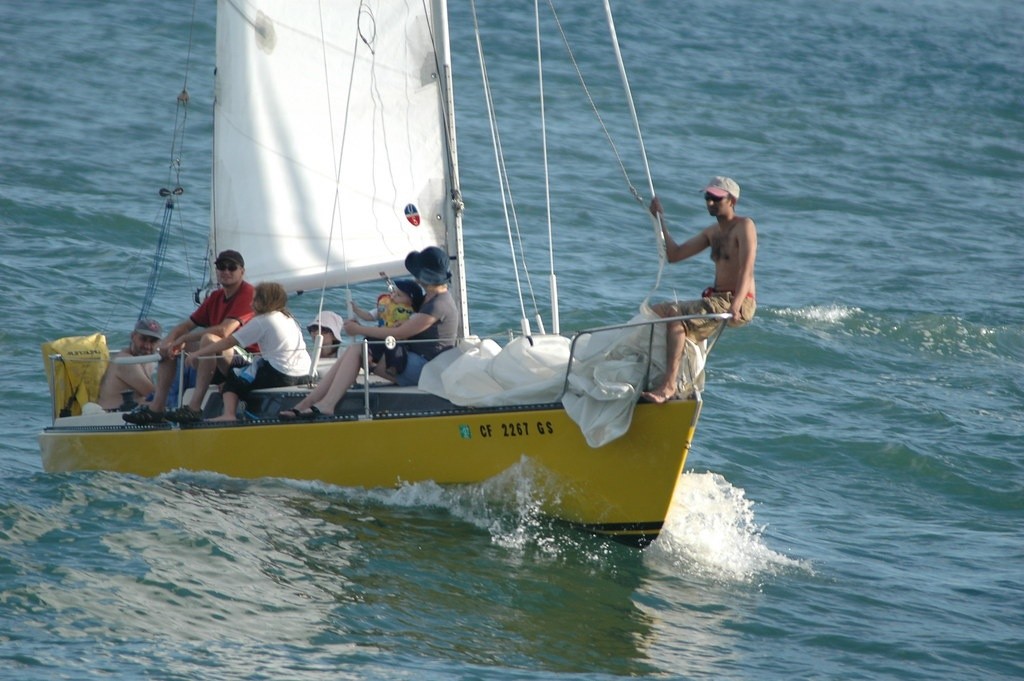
[276,244,458,420]
[641,177,758,404]
[96,249,344,424]
[351,278,423,378]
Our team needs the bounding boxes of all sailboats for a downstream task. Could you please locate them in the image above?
[38,0,734,548]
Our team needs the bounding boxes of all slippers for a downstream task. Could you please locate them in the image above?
[278,408,304,419]
[299,406,333,417]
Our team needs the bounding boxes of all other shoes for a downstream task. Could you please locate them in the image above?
[386,366,397,377]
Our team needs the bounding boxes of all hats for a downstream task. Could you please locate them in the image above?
[404,245,452,286]
[393,278,423,305]
[305,309,343,345]
[699,175,740,202]
[134,319,164,340]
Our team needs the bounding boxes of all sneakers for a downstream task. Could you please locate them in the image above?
[122,404,167,424]
[165,405,205,420]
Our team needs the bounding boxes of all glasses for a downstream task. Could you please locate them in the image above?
[216,266,239,271]
[308,325,330,334]
[703,193,729,203]
[213,250,245,268]
